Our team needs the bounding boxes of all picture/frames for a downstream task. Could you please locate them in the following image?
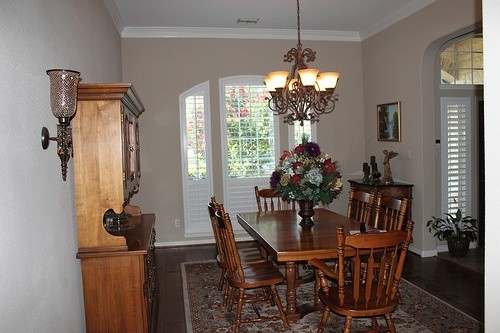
[377,101,401,142]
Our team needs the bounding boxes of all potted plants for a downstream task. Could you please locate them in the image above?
[426,208,482,258]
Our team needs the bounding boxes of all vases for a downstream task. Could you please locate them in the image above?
[298,200,316,225]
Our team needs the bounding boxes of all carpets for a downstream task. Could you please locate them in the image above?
[181,259,485,333]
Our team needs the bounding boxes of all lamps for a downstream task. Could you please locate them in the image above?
[263,0,340,125]
[40,69,81,181]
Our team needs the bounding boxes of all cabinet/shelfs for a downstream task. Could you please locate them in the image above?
[70,83,160,333]
[347,178,414,230]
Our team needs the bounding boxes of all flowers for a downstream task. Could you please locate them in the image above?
[270,133,344,205]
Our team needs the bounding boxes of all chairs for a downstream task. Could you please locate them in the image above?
[206,196,291,333]
[307,220,416,333]
[254,186,307,269]
[332,187,408,305]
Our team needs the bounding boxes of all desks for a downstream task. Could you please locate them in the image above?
[237,208,408,319]
